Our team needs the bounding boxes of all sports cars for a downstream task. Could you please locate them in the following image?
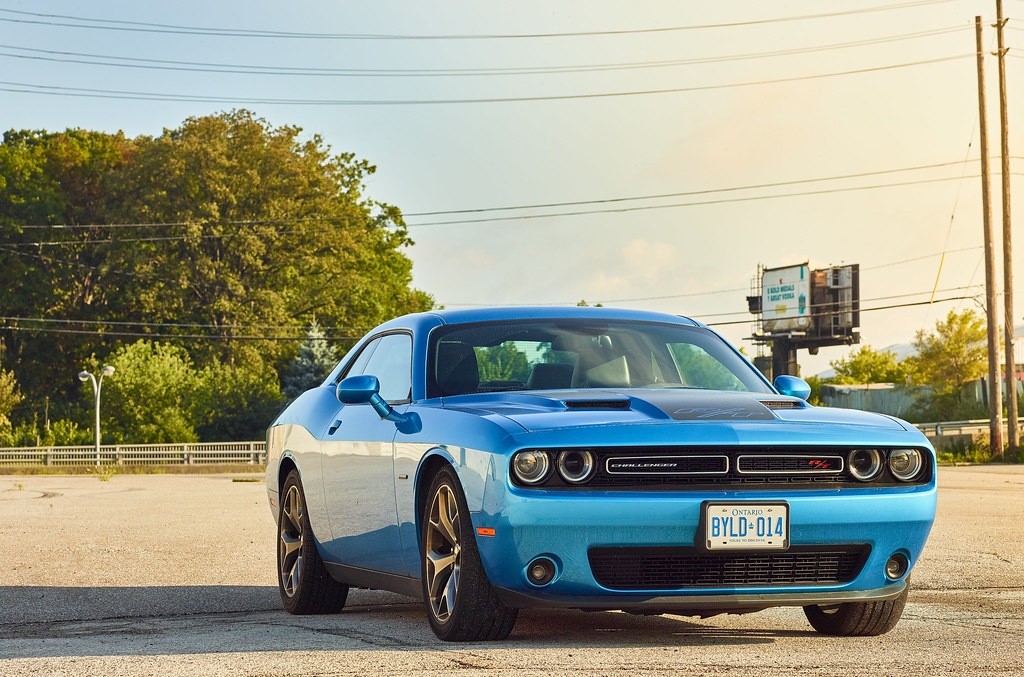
[264,304,939,644]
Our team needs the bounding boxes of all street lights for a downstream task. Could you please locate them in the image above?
[77,366,116,465]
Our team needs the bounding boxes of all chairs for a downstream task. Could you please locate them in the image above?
[570,349,630,388]
[526,360,574,389]
[436,342,479,395]
